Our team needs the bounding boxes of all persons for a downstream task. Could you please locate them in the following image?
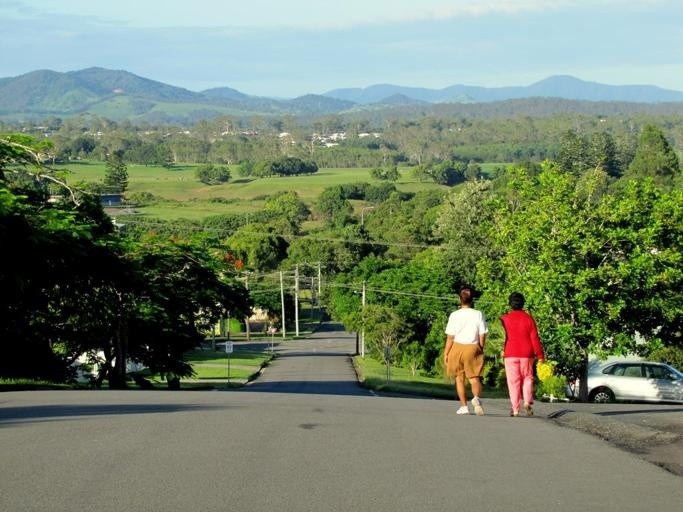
[440,285,489,415]
[497,291,546,419]
[534,355,576,400]
[86,356,106,389]
[575,339,590,402]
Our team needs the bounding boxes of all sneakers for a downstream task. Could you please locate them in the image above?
[507,411,520,416]
[455,405,469,416]
[471,395,484,416]
[523,402,535,416]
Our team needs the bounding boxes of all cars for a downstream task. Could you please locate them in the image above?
[564,359,682,405]
